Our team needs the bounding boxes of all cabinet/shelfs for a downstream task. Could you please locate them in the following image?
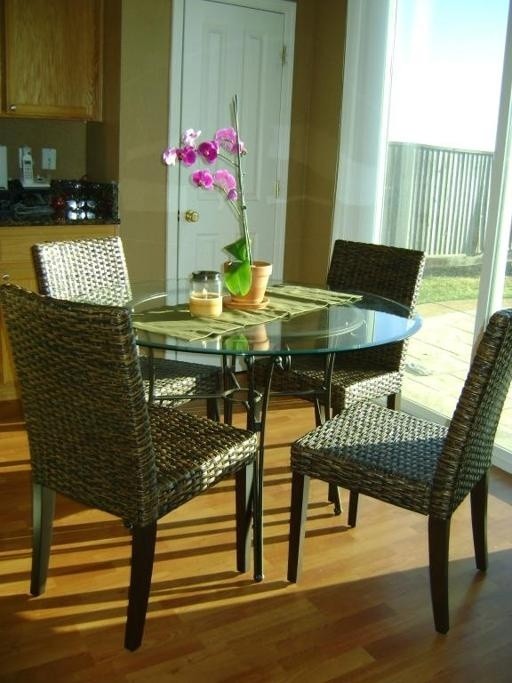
[1,0,121,429]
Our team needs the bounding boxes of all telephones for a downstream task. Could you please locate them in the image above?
[19,146,51,188]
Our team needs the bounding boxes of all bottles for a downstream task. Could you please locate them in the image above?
[188,271,223,318]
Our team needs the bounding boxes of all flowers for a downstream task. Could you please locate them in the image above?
[160,92,254,297]
